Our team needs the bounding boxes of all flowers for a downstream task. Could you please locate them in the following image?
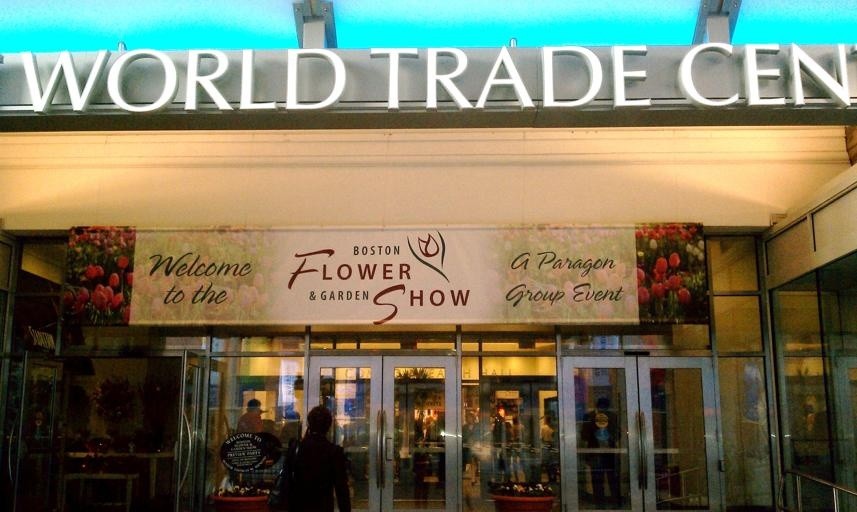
[60,225,136,325]
[211,484,270,496]
[488,481,554,497]
[634,222,709,323]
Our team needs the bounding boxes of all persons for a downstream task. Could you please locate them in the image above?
[408,406,555,508]
[282,404,351,511]
[236,398,265,486]
[580,395,622,510]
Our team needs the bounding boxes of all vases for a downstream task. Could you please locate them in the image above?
[491,495,558,512]
[210,495,270,512]
